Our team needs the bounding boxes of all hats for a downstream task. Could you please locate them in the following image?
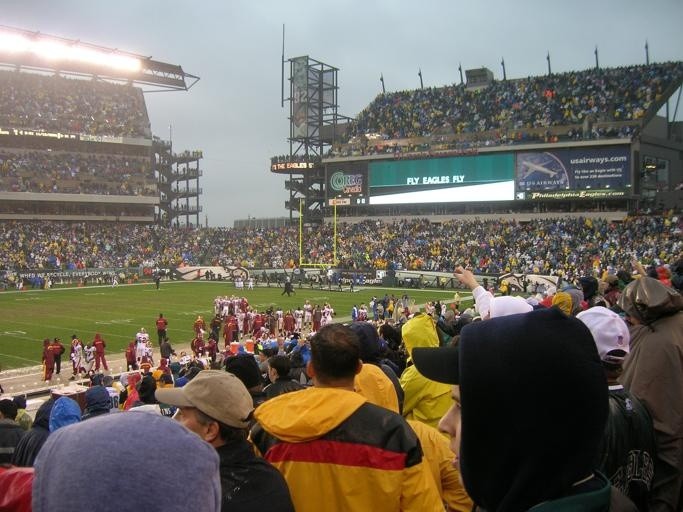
[155,370,256,429]
[575,306,631,365]
[413,347,459,384]
[604,275,618,283]
[225,354,262,388]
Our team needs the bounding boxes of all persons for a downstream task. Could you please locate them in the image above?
[1,60,683,511]
